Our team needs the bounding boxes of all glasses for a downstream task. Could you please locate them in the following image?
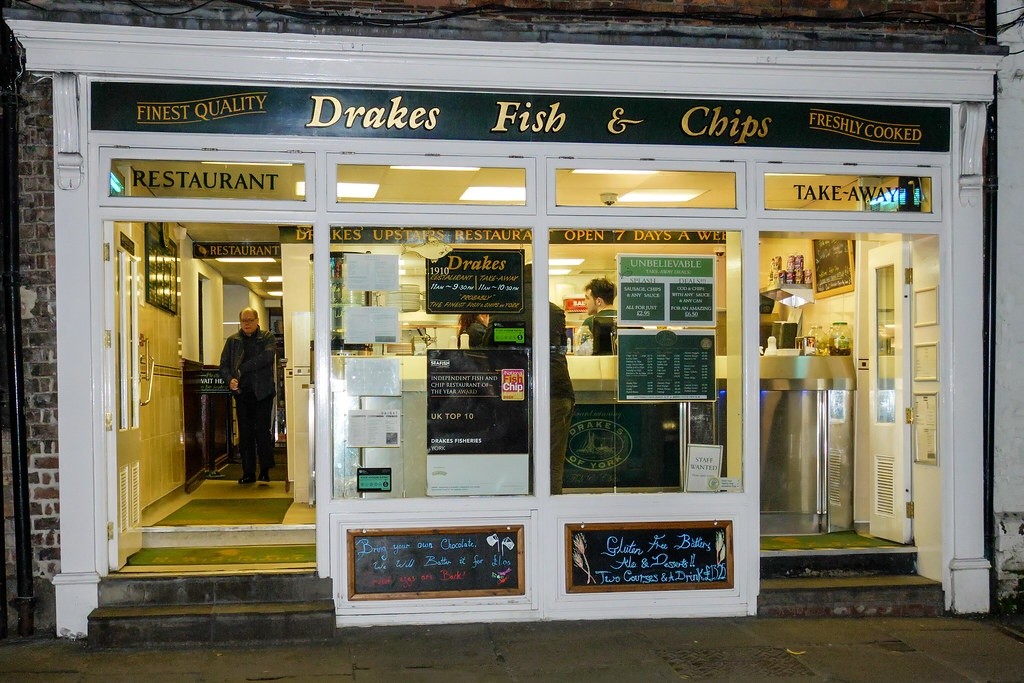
[239,317,257,325]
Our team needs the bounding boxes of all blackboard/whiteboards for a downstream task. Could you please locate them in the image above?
[810,237,854,300]
[564,522,736,593]
[343,523,527,602]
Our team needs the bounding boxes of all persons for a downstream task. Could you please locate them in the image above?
[577,276,620,357]
[454,311,492,351]
[482,262,575,496]
[218,307,277,485]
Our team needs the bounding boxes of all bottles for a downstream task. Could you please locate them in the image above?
[768,336,777,356]
[574,324,595,356]
[448,330,458,348]
[459,329,471,349]
[807,321,852,356]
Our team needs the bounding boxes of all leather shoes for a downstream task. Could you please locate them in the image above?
[259,471,269,482]
[239,474,256,484]
